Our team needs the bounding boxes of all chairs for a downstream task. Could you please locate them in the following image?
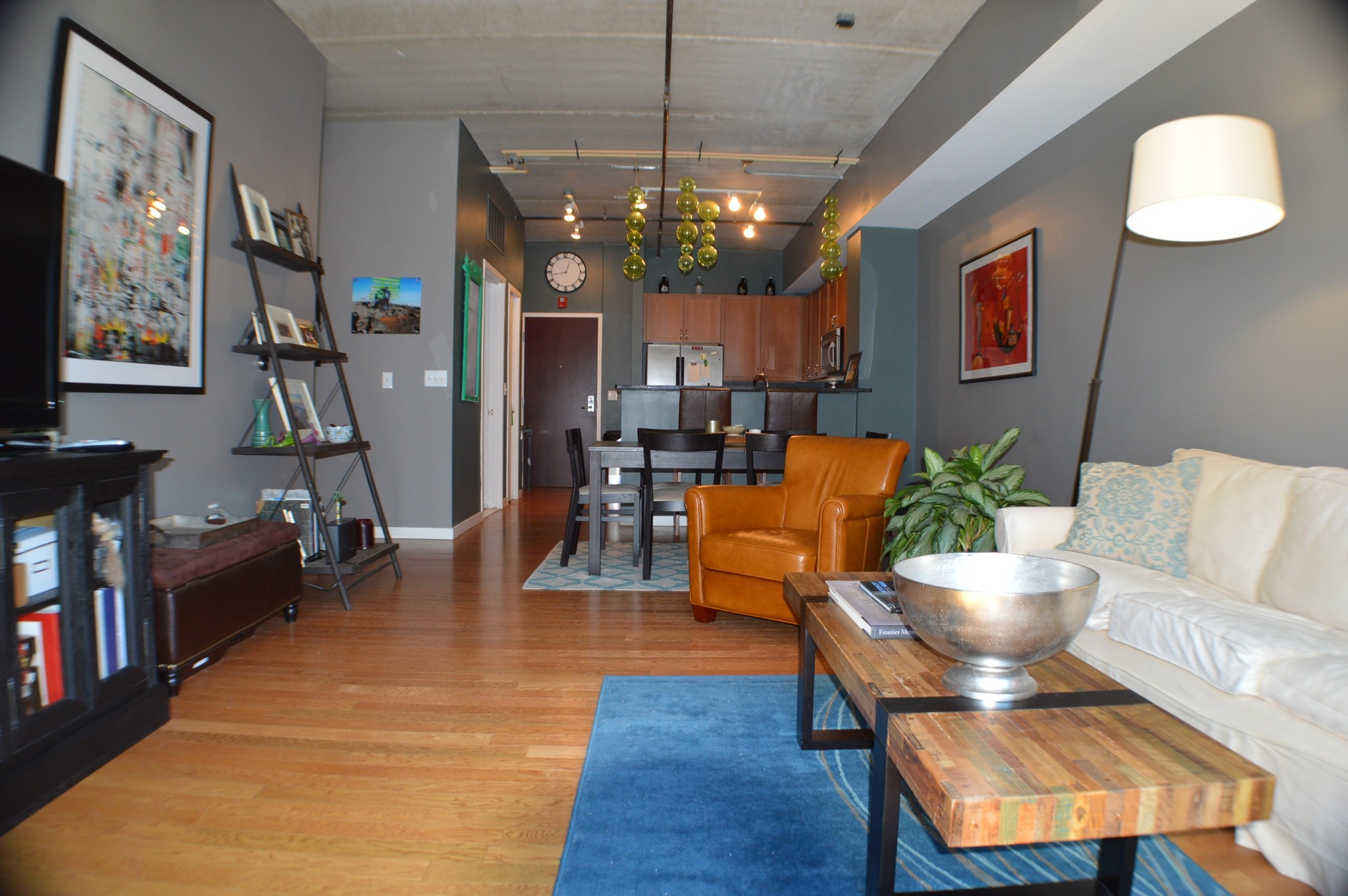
[745,432,832,486]
[755,388,821,487]
[559,427,644,568]
[670,387,735,487]
[633,427,730,581]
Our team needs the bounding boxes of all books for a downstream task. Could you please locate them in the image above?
[12,581,132,720]
[859,580,904,614]
[825,579,919,641]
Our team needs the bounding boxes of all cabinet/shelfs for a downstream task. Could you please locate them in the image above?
[642,265,850,384]
[0,436,177,845]
[219,158,405,612]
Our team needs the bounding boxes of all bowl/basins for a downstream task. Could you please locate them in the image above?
[326,425,353,442]
[723,425,746,436]
[891,551,1099,702]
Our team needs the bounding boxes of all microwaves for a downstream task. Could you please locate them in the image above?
[820,330,844,373]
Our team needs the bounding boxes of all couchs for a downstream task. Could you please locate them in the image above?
[681,436,911,635]
[992,445,1348,895]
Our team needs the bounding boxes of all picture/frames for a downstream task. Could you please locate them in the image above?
[293,318,325,349]
[238,183,281,246]
[842,351,863,390]
[261,302,303,346]
[955,227,1039,385]
[459,255,485,403]
[284,207,313,261]
[251,312,268,345]
[46,12,217,397]
[267,375,328,444]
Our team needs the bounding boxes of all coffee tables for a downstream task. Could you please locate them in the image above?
[778,569,1275,896]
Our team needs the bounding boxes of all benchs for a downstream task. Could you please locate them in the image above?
[116,521,308,701]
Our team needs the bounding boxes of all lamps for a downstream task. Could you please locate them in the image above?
[742,222,755,238]
[570,220,585,240]
[753,203,766,222]
[1066,113,1289,504]
[727,192,741,212]
[563,203,576,222]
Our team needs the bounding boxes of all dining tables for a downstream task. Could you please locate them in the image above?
[585,436,819,576]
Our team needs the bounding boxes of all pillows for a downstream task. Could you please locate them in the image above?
[1057,454,1204,580]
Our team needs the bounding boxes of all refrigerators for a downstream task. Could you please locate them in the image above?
[646,343,723,386]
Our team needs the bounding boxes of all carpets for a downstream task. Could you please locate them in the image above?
[549,670,1241,896]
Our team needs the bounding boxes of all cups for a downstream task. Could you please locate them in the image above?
[749,429,761,433]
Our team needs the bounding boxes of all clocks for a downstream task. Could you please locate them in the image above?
[545,251,587,293]
